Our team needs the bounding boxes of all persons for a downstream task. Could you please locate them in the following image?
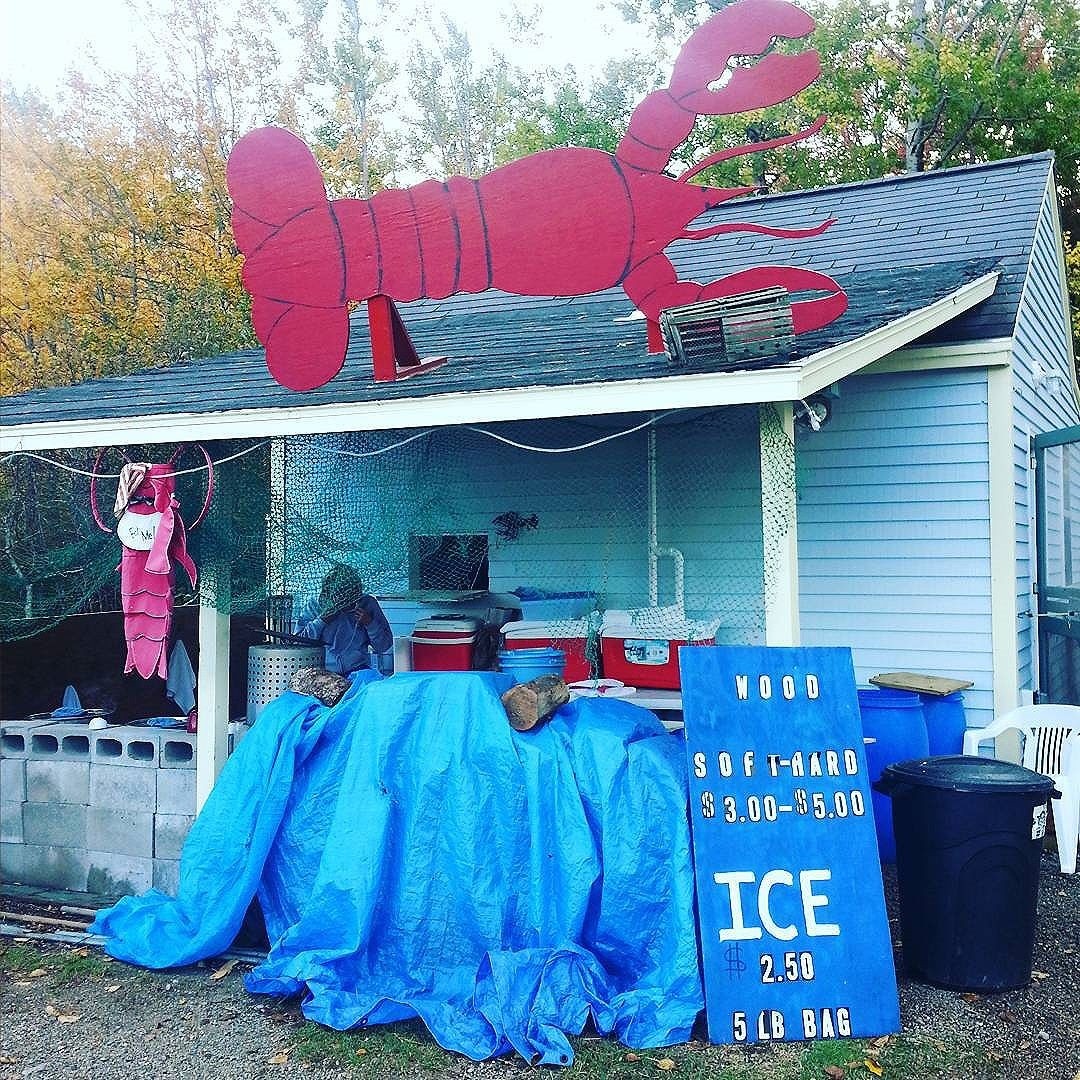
[291,567,394,679]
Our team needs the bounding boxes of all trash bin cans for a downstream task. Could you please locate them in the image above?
[872,756,1062,995]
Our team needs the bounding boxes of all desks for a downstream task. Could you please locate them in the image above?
[617,688,683,710]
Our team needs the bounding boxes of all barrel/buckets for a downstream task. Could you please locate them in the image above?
[856,688,930,863]
[879,690,966,757]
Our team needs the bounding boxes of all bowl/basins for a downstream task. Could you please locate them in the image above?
[497,646,567,684]
[88,718,108,729]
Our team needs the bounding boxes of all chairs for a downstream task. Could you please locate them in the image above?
[962,705,1080,873]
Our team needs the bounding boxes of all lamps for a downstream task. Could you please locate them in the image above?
[492,511,538,539]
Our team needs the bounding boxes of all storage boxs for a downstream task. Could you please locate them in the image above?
[504,626,594,684]
[408,617,481,671]
[601,620,720,689]
